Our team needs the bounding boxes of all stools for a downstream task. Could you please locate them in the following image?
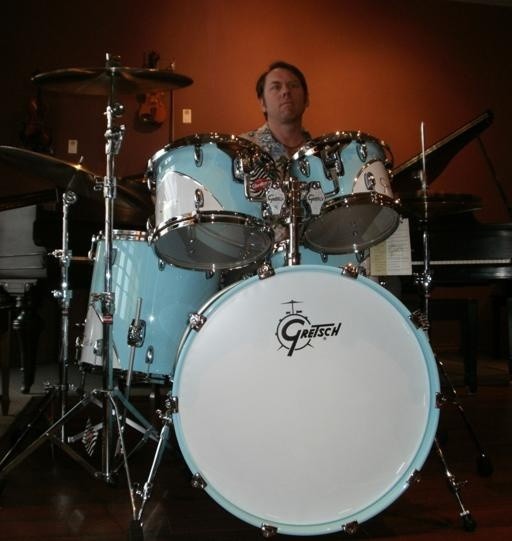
[429,299,478,386]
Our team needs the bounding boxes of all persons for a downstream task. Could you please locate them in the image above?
[237,59,317,167]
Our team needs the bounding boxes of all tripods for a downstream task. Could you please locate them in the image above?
[0,94,480,536]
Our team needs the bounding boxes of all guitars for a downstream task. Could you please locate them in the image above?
[137,51,168,126]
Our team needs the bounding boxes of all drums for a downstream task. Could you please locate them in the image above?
[78,229,223,383]
[288,131,401,254]
[145,132,280,275]
[172,265,441,536]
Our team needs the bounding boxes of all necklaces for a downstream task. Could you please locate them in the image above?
[271,131,307,151]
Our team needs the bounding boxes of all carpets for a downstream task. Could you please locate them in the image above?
[0,396,207,505]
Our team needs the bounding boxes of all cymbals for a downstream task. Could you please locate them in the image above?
[31,68,193,94]
[1,145,143,208]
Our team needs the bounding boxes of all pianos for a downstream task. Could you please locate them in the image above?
[1,188,103,395]
[370,112,512,396]
